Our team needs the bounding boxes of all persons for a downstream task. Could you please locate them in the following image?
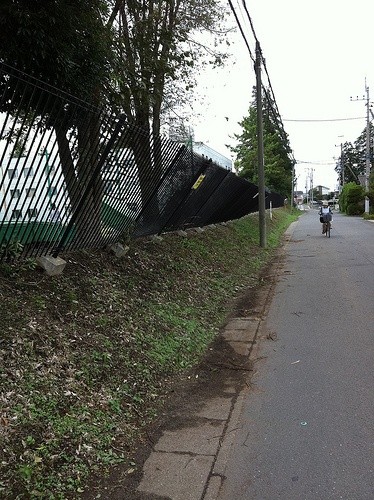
[318,199,334,234]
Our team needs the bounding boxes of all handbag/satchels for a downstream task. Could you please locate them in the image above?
[320,216,326,223]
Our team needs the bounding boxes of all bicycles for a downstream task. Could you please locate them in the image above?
[318,211,332,238]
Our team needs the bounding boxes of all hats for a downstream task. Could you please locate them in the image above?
[323,201,328,206]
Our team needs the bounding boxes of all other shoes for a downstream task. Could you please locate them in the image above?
[322,231,326,234]
